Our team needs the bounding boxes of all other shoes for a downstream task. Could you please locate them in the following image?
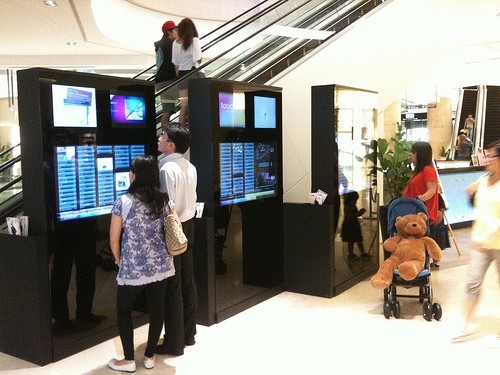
[185,336,195,345]
[348,253,360,260]
[360,252,372,258]
[108,358,136,372]
[154,345,184,355]
[144,356,155,368]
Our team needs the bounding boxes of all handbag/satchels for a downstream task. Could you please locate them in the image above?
[162,201,188,255]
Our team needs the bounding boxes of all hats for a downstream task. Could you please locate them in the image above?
[162,21,177,33]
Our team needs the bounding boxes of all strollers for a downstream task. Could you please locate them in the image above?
[215,188,235,275]
[96,228,125,271]
[383,198,442,321]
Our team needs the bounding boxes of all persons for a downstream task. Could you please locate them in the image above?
[41,127,103,332]
[401,142,438,289]
[154,21,179,131]
[334,165,372,260]
[430,193,451,268]
[108,155,176,373]
[456,128,470,145]
[171,18,205,126]
[464,114,475,140]
[452,140,500,343]
[157,122,197,355]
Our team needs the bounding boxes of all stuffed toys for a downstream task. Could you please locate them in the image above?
[369,212,443,289]
